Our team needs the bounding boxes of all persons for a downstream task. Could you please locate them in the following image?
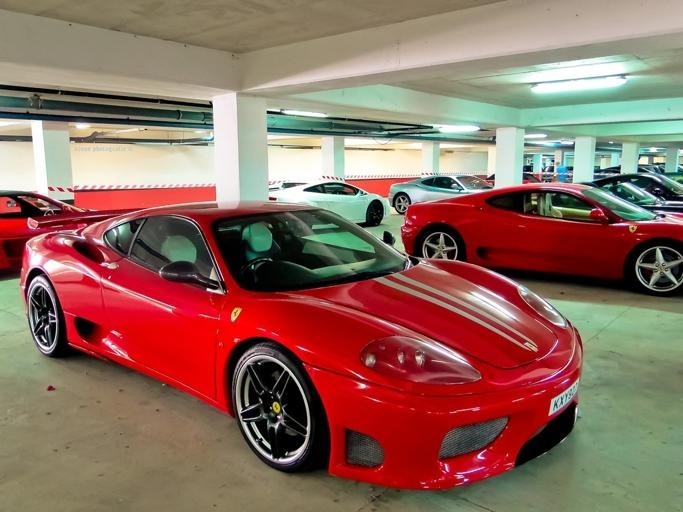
[554,162,560,172]
[556,165,569,182]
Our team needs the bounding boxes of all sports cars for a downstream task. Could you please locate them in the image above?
[268,178,389,233]
[18,200,583,495]
[399,162,683,296]
[0,189,146,280]
[389,173,495,214]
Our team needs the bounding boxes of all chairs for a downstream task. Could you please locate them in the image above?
[160,235,196,266]
[537,195,548,216]
[241,222,273,261]
[546,193,563,218]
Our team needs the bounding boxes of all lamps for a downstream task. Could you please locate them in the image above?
[530,75,626,94]
[280,110,328,118]
